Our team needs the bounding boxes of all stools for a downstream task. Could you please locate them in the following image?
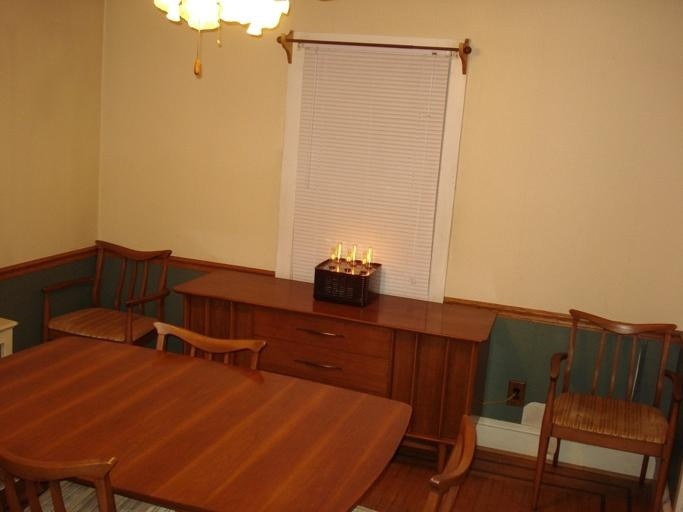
[0,317,18,358]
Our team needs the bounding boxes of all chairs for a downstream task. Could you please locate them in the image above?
[152,318,266,369]
[41,239,173,345]
[426,416,478,509]
[529,308,678,510]
[1,446,118,510]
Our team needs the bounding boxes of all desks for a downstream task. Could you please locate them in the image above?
[1,335,413,510]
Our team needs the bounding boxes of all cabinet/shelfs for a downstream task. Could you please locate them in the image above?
[173,268,497,475]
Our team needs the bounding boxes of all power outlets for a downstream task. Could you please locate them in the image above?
[506,380,525,405]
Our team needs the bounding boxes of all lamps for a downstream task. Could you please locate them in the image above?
[150,0,290,78]
